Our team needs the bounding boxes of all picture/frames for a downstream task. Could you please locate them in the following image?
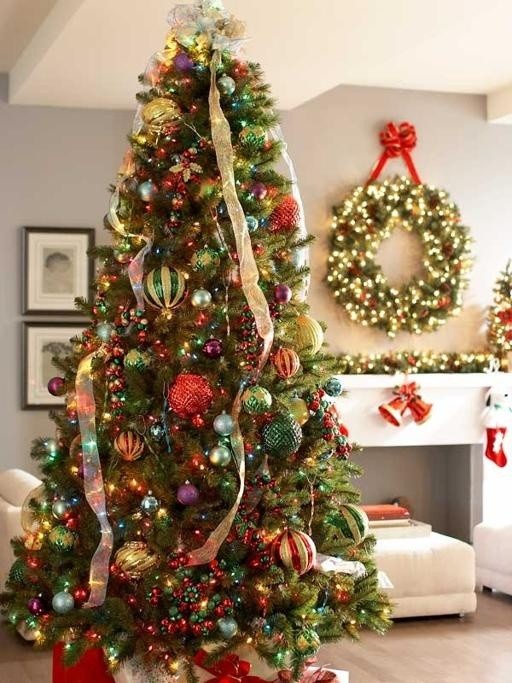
[18,320,93,411]
[21,224,95,316]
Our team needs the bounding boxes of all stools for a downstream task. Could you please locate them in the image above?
[359,504,479,620]
[473,518,512,599]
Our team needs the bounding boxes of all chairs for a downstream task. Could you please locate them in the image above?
[1,468,45,596]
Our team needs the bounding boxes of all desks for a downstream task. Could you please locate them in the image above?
[49,637,115,683]
